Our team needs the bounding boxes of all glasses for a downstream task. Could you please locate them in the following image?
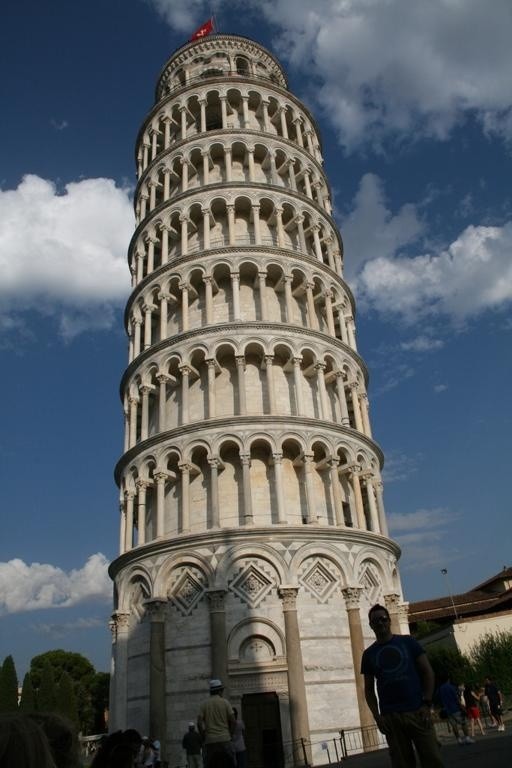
[371,617,390,625]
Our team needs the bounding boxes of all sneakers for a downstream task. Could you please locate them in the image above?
[456,722,505,745]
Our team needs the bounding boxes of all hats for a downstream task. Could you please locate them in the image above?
[208,679,226,690]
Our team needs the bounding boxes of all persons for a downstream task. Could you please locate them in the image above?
[88,727,162,767]
[197,678,237,767]
[438,674,507,744]
[230,706,248,767]
[181,720,201,766]
[360,603,445,767]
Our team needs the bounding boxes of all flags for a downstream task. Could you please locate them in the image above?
[191,15,214,42]
